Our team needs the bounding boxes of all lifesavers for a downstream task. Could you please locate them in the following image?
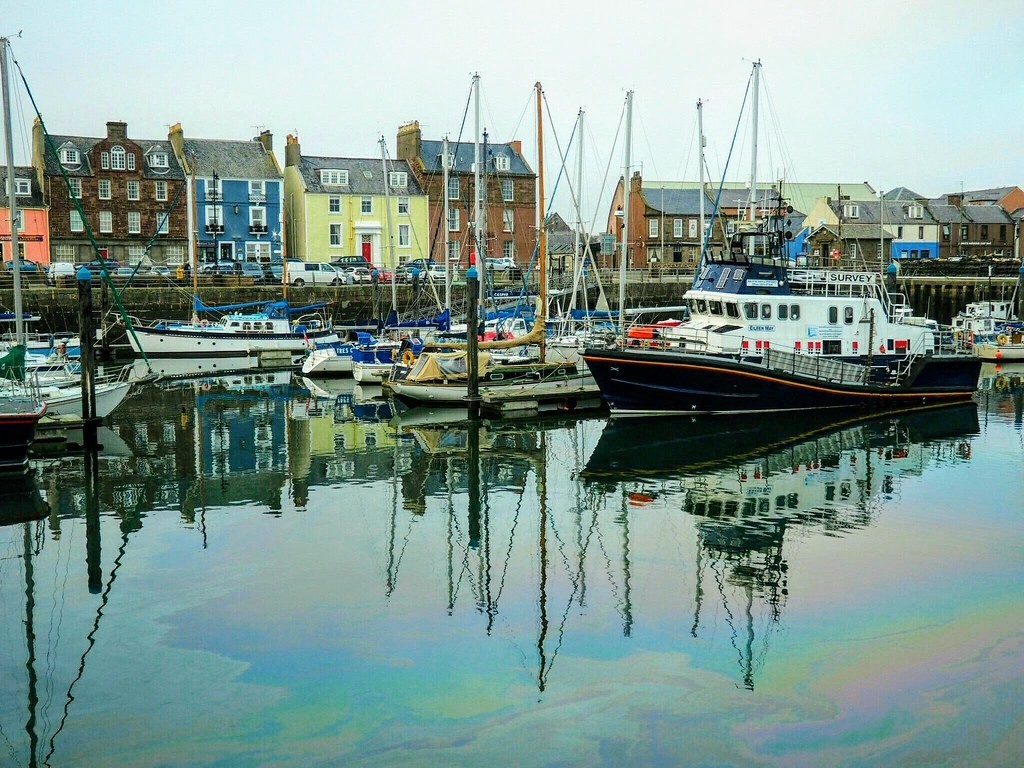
[392,349,396,360]
[402,351,414,363]
[997,334,1008,345]
[994,376,1007,389]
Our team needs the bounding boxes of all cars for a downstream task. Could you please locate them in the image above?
[329,255,370,270]
[197,258,304,285]
[372,266,398,284]
[344,266,371,284]
[395,257,446,283]
[74,258,120,275]
[773,256,804,267]
[45,261,76,285]
[486,257,516,271]
[6,260,51,272]
[145,265,171,275]
[110,268,140,276]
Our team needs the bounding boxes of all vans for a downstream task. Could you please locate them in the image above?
[281,262,347,287]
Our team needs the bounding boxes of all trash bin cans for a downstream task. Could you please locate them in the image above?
[177,267,185,279]
[1012,330,1022,344]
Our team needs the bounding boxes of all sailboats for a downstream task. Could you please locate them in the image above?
[0,34,983,433]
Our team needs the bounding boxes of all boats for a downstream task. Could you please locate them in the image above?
[976,362,1024,440]
[950,263,1024,363]
[0,374,974,767]
